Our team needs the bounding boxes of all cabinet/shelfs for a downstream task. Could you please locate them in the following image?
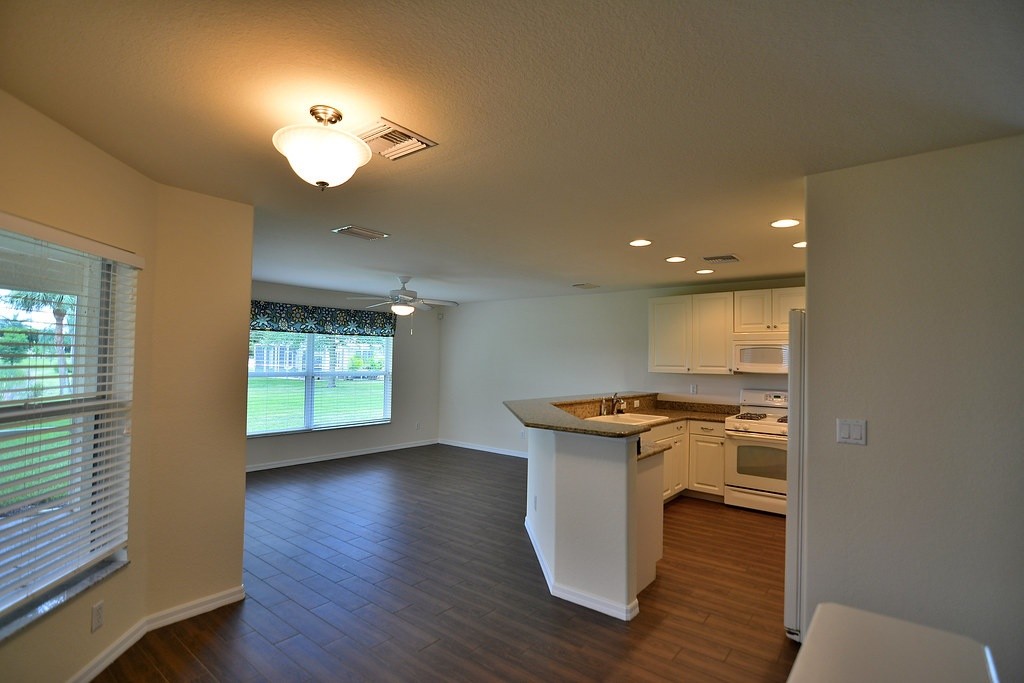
[687,418,725,504]
[647,286,742,376]
[652,417,689,503]
[733,285,806,334]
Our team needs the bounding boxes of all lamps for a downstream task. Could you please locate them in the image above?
[391,302,415,316]
[272,104,373,192]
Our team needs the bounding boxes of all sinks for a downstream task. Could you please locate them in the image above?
[585,413,669,426]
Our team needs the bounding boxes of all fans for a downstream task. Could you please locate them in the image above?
[347,272,459,313]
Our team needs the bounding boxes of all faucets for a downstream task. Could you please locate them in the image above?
[611,391,625,415]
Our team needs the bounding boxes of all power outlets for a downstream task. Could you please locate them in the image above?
[621,402,626,409]
[91,600,103,634]
[633,400,640,408]
